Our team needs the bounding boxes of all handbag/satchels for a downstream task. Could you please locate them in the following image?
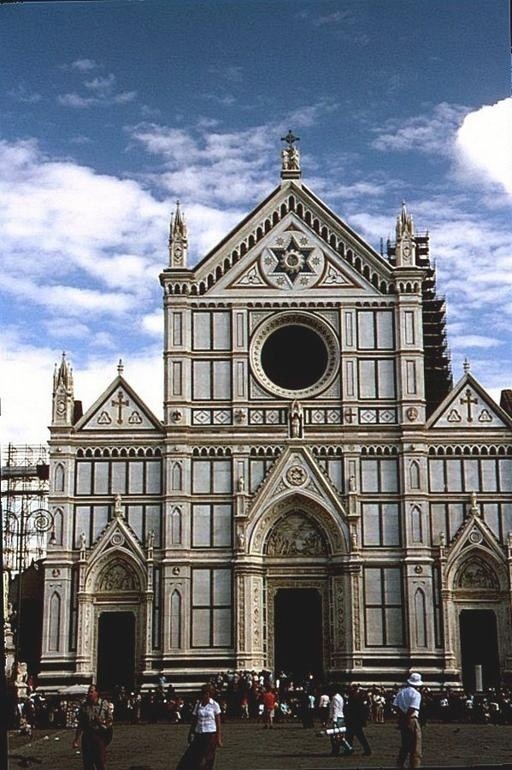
[100,727,112,746]
[330,733,345,746]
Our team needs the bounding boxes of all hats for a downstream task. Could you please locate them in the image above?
[407,673,424,685]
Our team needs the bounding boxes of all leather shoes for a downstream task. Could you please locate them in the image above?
[331,749,354,756]
[362,751,372,756]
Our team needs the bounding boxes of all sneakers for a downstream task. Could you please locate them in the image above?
[264,725,273,729]
